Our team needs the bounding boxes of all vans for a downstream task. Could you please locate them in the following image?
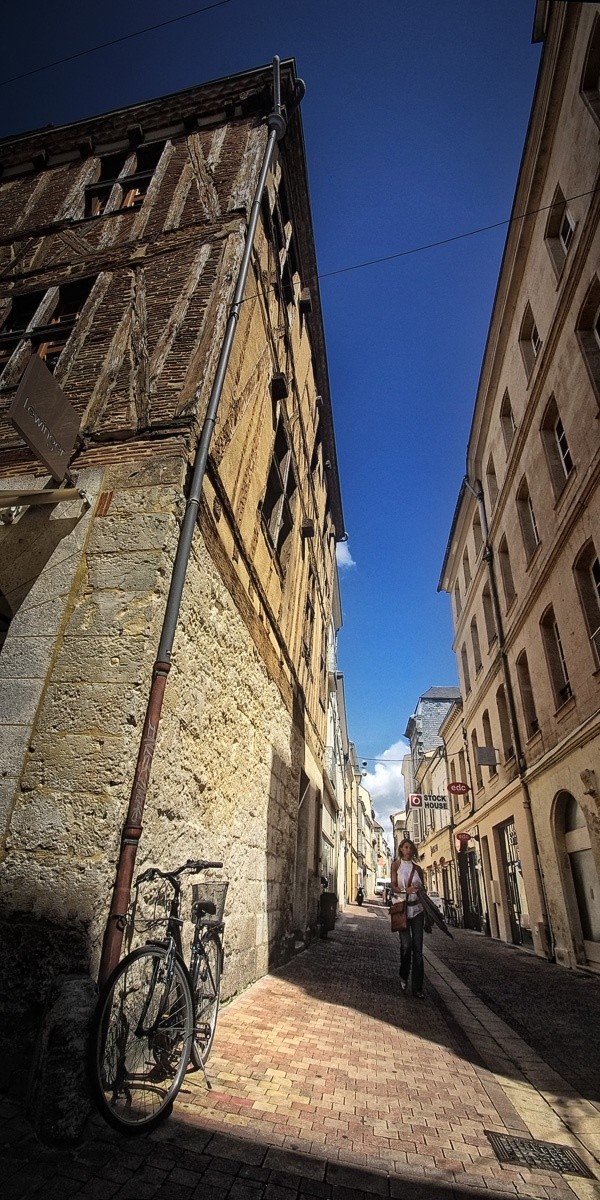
[375,877,392,894]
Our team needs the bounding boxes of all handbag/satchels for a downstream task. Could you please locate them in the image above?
[388,901,407,933]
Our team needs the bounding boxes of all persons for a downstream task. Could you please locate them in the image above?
[390,839,426,1000]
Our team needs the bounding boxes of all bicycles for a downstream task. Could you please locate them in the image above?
[88,860,231,1136]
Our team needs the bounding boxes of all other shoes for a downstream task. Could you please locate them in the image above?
[400,980,408,989]
[415,991,424,999]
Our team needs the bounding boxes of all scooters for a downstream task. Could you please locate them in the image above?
[355,886,364,906]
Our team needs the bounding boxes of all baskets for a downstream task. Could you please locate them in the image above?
[192,880,229,925]
